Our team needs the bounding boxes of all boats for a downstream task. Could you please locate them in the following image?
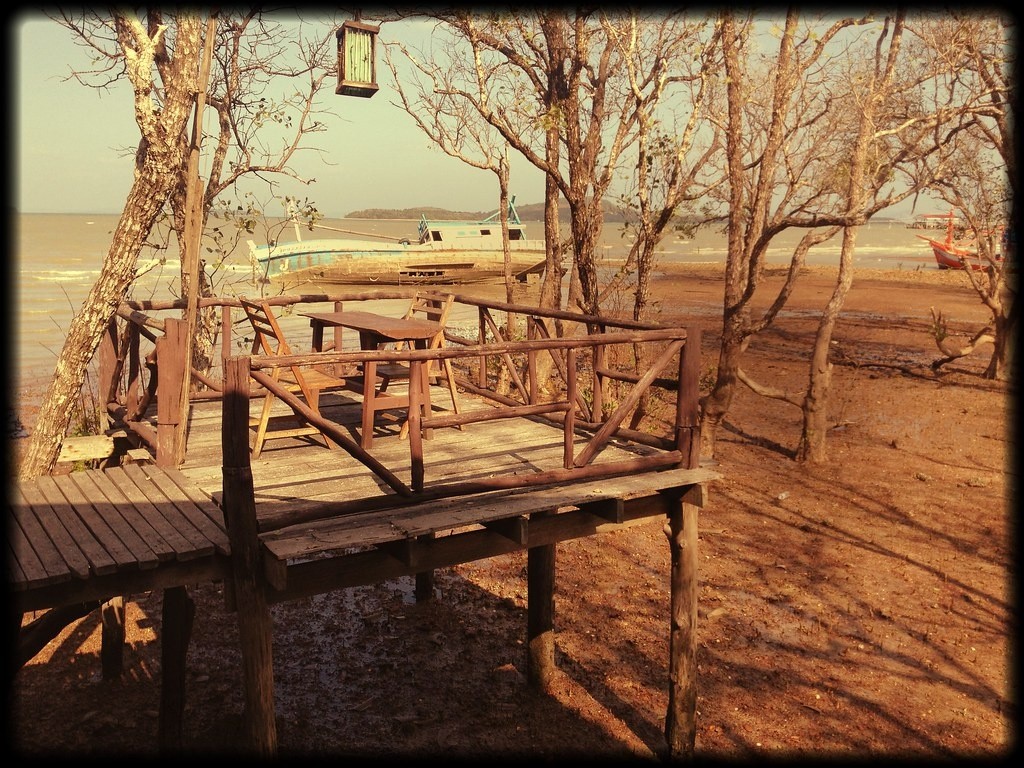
[245,193,548,285]
[915,208,1015,274]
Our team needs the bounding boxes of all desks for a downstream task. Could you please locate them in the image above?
[298,309,446,440]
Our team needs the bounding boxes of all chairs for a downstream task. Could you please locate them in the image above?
[355,291,466,440]
[237,294,346,458]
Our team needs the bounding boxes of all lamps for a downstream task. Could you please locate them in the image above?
[336,12,380,95]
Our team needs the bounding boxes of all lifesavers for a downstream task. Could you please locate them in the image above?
[398,239,411,245]
